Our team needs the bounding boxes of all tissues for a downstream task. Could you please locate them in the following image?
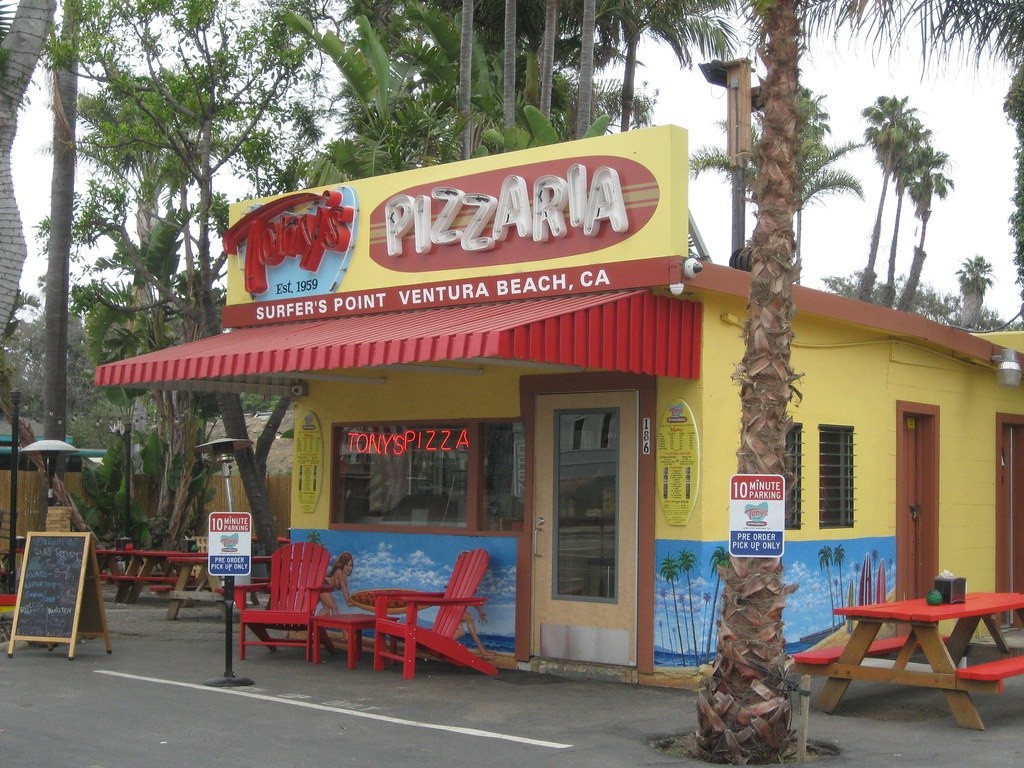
[115,535,133,550]
[933,569,966,603]
[180,535,197,553]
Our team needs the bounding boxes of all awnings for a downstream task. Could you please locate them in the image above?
[94,289,701,387]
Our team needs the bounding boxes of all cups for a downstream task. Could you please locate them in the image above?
[927,589,942,605]
[125,544,133,550]
[191,545,198,552]
[16,537,24,548]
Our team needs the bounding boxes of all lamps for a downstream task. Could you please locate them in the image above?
[992,348,1022,388]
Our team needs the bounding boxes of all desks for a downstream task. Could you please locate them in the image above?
[167,556,273,621]
[95,549,207,603]
[310,614,399,669]
[815,593,1024,730]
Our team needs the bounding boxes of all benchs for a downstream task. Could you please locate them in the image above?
[99,574,270,593]
[793,634,949,665]
[955,655,1024,681]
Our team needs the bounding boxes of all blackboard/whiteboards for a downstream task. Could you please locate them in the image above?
[10,531,111,643]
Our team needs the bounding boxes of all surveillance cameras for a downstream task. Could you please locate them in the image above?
[291,385,303,395]
[682,257,704,279]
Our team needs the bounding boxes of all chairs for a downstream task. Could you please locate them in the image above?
[221,543,334,662]
[372,549,498,680]
